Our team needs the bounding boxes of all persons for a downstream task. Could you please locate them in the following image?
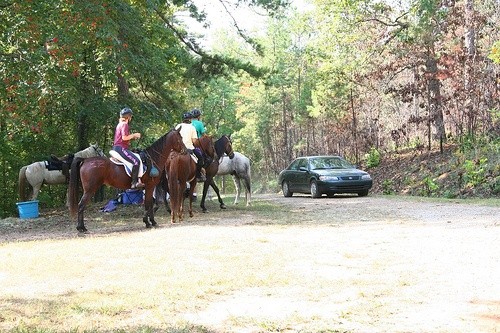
[191,109,207,139]
[113,108,141,189]
[175,113,206,182]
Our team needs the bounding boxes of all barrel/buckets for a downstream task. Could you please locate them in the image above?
[16,200,39,220]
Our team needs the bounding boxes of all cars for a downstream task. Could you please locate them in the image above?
[278,155,373,199]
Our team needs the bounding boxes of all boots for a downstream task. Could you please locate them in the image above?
[197,156,204,179]
[130,164,141,190]
[200,159,211,180]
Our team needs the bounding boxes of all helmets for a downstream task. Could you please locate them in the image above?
[191,109,201,117]
[182,112,195,121]
[120,107,134,116]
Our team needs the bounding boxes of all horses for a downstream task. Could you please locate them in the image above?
[68,125,187,232]
[167,135,214,223]
[201,133,235,209]
[19,142,107,210]
[216,151,253,207]
